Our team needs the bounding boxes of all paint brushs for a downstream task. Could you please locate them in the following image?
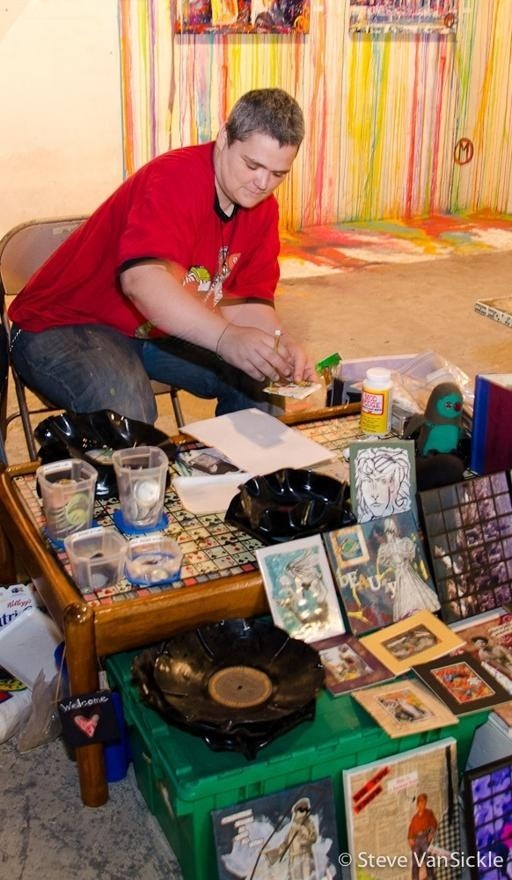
[269,330,282,388]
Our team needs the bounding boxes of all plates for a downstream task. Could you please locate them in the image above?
[32,407,180,502]
[128,615,327,757]
[220,463,359,550]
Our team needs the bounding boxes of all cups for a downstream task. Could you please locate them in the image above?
[110,444,171,530]
[34,457,99,541]
[62,524,129,594]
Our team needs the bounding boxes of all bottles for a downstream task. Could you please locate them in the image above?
[358,366,395,437]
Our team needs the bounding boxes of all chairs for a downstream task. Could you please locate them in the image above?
[0,214,187,460]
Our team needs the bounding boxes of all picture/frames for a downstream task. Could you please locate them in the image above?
[252,438,512,880]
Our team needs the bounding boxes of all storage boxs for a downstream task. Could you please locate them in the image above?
[107,646,494,880]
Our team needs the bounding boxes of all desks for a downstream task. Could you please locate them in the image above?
[1,398,436,808]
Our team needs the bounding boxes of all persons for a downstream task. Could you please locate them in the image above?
[376,518,439,617]
[471,636,512,674]
[264,798,319,880]
[8,89,321,429]
[404,792,442,879]
[356,449,412,522]
[277,549,328,632]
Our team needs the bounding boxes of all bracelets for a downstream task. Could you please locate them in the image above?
[213,322,232,361]
